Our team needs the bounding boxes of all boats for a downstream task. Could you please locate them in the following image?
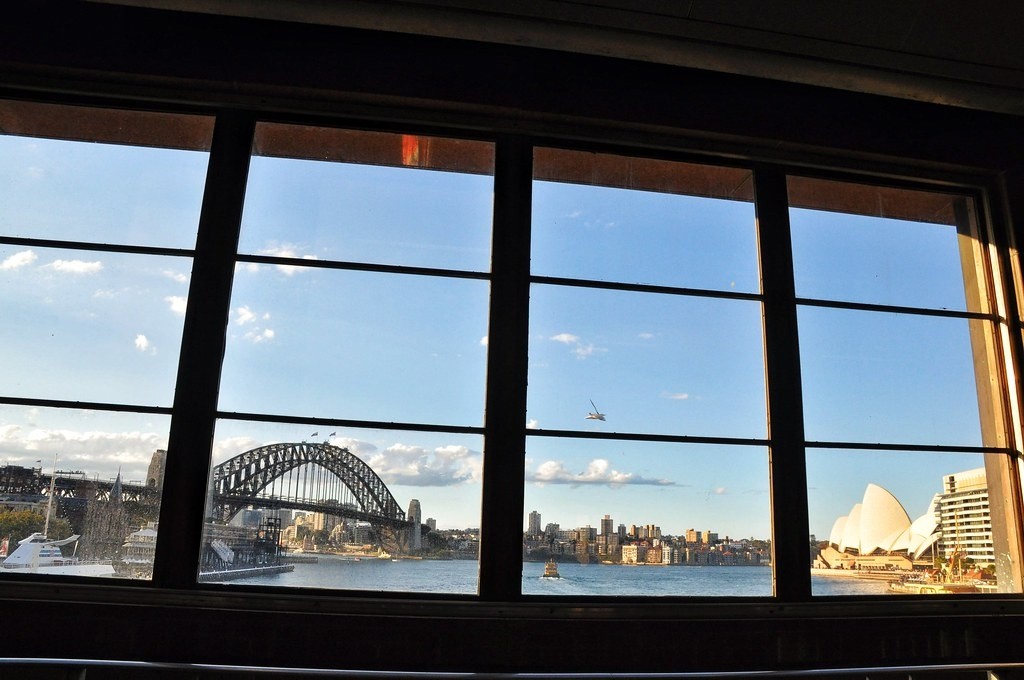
[0,450,116,579]
[541,556,562,579]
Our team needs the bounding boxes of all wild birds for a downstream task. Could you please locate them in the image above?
[585,399,606,421]
